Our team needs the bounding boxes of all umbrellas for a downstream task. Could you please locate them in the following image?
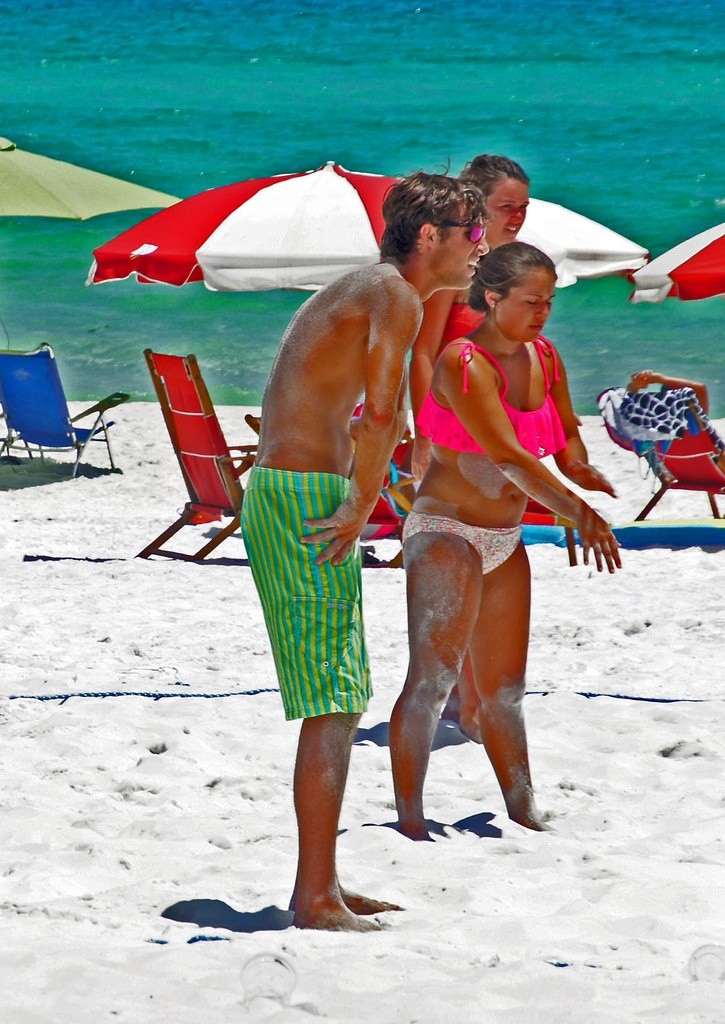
[0,135,184,221]
[83,161,649,293]
[626,221,725,304]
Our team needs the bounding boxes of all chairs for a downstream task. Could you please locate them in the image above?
[596,385,725,521]
[134,348,257,562]
[244,414,261,436]
[0,341,116,478]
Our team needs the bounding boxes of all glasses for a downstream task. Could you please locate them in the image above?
[433,219,486,244]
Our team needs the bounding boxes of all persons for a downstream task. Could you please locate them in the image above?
[409,154,530,744]
[626,369,710,418]
[391,242,623,841]
[239,171,489,934]
